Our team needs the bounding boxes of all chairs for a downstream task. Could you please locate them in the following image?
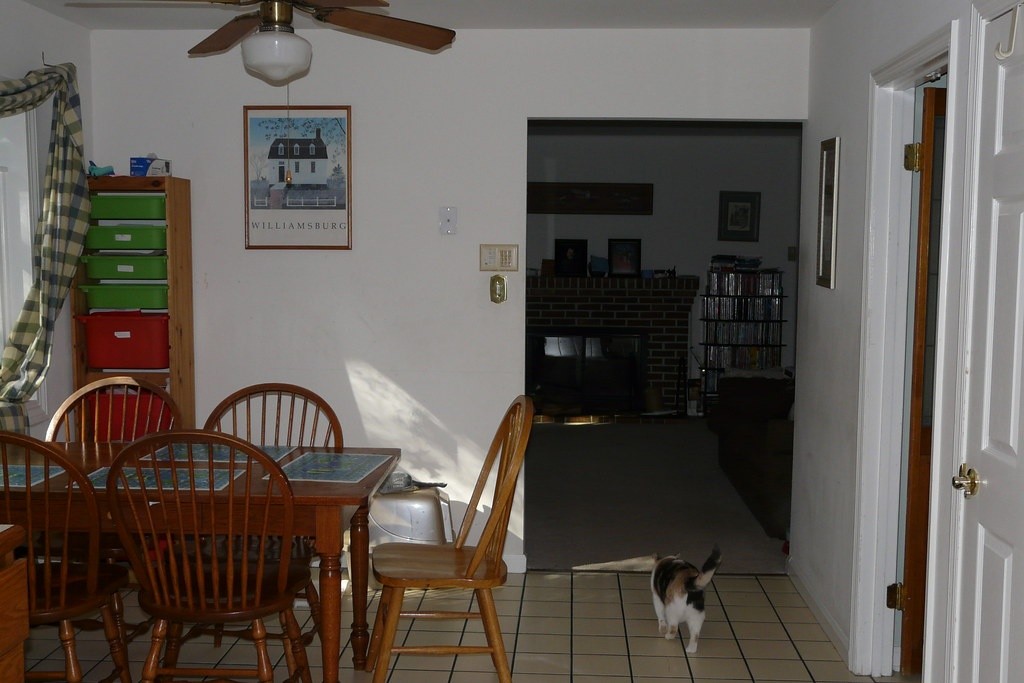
[368,395,534,683]
[0,375,340,683]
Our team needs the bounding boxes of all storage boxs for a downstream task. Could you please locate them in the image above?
[129,157,172,176]
[76,193,168,442]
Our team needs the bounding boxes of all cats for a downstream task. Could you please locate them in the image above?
[649,540,722,653]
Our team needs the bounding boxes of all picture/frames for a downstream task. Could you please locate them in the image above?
[243,105,353,250]
[718,190,761,242]
[555,238,588,277]
[608,238,642,277]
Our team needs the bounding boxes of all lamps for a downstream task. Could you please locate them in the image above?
[241,0,312,81]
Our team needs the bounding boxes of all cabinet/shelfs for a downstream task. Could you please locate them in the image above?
[68,175,196,437]
[699,267,788,416]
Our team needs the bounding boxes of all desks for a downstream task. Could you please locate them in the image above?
[0,439,401,683]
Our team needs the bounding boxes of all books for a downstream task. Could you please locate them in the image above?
[701,270,781,405]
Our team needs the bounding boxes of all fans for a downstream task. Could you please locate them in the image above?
[185,0,456,54]
